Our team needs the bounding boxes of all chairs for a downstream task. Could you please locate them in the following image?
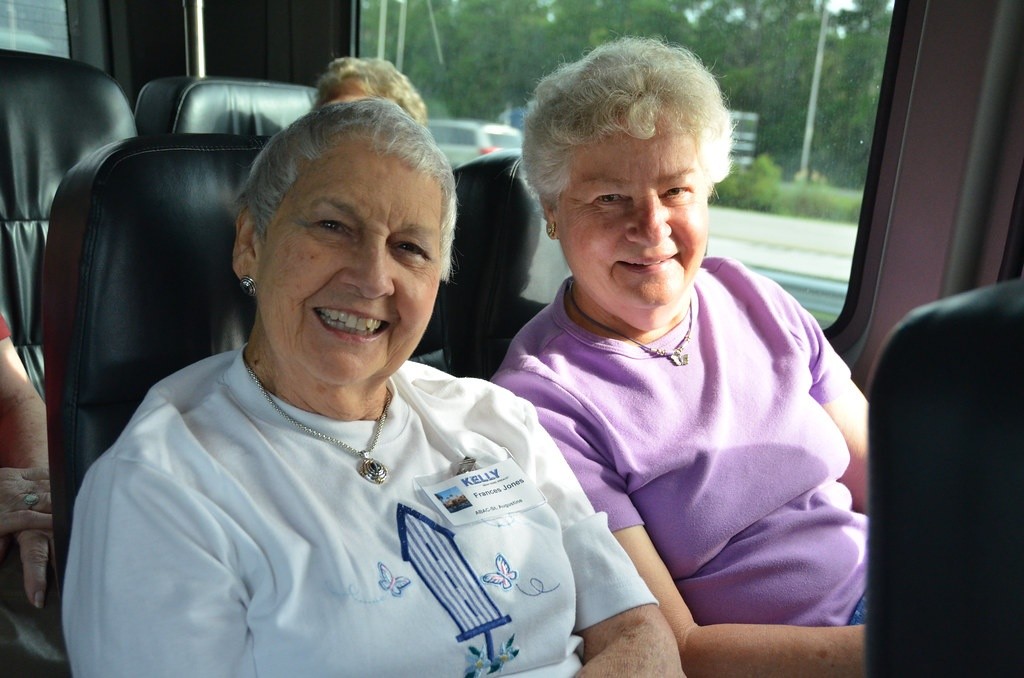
[0,50,1024,678]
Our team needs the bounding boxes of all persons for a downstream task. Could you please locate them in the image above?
[314,56,428,127]
[62,97,683,678]
[0,312,54,609]
[490,38,869,678]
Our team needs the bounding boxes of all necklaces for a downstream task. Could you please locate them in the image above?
[569,281,692,366]
[244,362,391,485]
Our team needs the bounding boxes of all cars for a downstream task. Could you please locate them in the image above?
[427,119,523,168]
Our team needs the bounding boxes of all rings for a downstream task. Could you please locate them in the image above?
[24,493,40,510]
